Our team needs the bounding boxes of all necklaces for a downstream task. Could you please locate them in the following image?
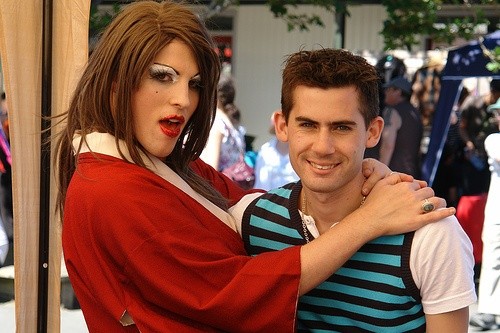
[300,189,365,243]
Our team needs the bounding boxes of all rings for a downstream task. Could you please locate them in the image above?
[422,198,435,213]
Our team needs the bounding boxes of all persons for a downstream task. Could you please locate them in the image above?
[227,49,474,332]
[372,46,449,122]
[191,65,302,190]
[63,0,456,332]
[185,72,500,329]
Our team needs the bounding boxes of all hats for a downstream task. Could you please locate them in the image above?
[382,76,412,96]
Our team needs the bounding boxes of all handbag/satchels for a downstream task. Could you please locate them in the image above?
[221,160,256,189]
